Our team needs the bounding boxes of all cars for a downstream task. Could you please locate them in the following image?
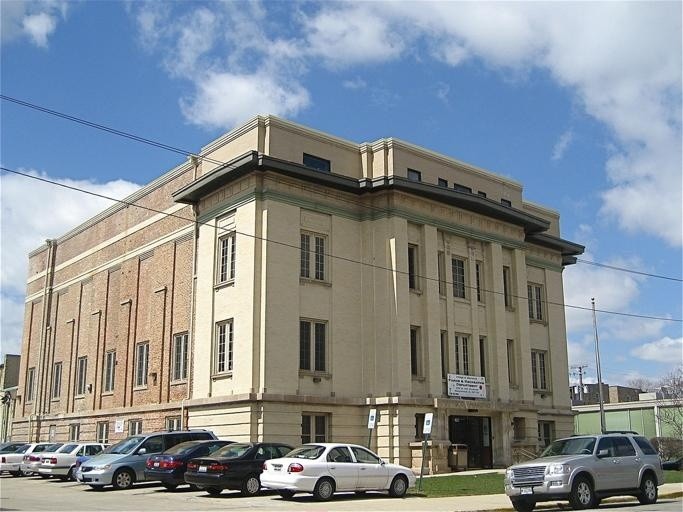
[145,439,416,502]
[1,442,113,480]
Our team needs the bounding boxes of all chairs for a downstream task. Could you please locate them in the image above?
[336,455,347,462]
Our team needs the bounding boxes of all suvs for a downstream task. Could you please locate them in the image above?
[505,431,664,511]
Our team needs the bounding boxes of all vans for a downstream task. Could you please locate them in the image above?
[78,430,218,490]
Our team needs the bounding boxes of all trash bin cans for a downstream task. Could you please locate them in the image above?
[448,444,467,471]
